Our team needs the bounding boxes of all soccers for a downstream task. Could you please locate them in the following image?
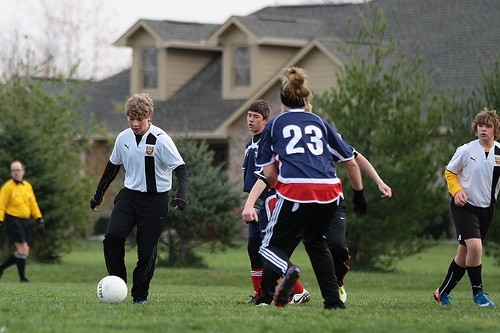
[97,276,128,303]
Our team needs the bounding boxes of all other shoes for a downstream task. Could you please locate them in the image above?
[433,288,451,306]
[273,265,301,308]
[338,284,347,304]
[246,295,258,304]
[472,290,494,307]
[323,299,346,309]
[287,288,311,305]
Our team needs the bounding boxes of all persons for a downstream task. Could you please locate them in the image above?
[90,94,188,304]
[0,160,44,282]
[241,135,392,306]
[254,67,368,310]
[433,109,500,306]
[242,100,310,305]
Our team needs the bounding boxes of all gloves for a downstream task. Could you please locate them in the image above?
[351,188,368,217]
[36,218,46,237]
